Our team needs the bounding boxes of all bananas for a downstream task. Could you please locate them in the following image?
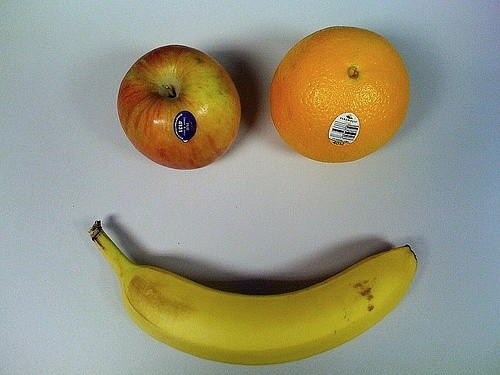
[88,220,418,366]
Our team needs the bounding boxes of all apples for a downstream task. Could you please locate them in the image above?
[117,44,242,170]
